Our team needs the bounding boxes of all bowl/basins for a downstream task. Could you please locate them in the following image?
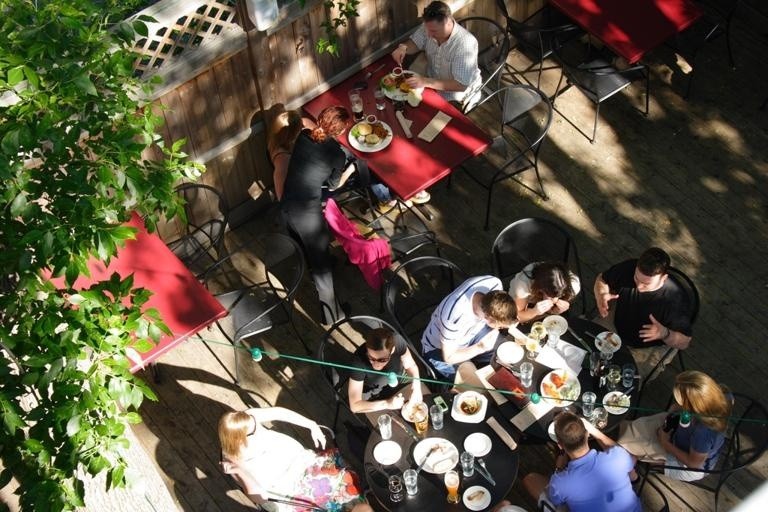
[457,390,483,416]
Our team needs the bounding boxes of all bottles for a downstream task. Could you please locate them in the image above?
[593,359,608,391]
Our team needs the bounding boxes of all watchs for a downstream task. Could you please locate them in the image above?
[664,326,669,340]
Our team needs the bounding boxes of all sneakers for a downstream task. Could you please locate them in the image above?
[410,190,431,204]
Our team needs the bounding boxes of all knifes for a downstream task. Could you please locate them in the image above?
[473,464,496,486]
[391,417,419,443]
[568,327,592,353]
[415,449,434,475]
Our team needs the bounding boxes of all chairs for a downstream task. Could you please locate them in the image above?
[454,16,509,120]
[202,233,304,385]
[324,199,444,313]
[495,0,574,93]
[382,254,472,357]
[490,217,587,319]
[684,0,736,101]
[589,267,700,412]
[637,393,768,511]
[221,423,336,510]
[318,316,436,443]
[140,184,233,292]
[447,82,552,234]
[552,28,650,144]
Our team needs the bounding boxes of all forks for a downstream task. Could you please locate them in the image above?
[478,459,492,477]
[496,360,521,378]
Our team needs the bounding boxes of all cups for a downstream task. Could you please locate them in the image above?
[388,475,404,502]
[414,416,428,434]
[589,352,600,375]
[348,90,360,104]
[582,391,597,417]
[526,334,539,360]
[377,414,392,440]
[591,407,609,429]
[530,322,546,339]
[519,362,534,388]
[352,98,364,122]
[403,469,418,496]
[374,91,386,110]
[600,351,613,369]
[444,470,461,504]
[622,364,635,388]
[430,405,444,430]
[460,452,474,477]
[607,365,620,391]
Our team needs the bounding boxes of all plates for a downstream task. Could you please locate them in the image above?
[450,391,488,424]
[540,369,581,407]
[496,341,525,364]
[372,440,402,465]
[602,391,630,414]
[547,421,558,443]
[382,70,424,101]
[594,331,621,352]
[401,400,428,422]
[412,437,459,474]
[462,485,492,511]
[463,432,492,457]
[348,119,393,153]
[542,315,568,336]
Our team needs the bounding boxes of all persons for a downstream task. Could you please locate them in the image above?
[490,472,567,512]
[218,407,361,512]
[267,110,303,203]
[391,1,482,115]
[508,260,581,323]
[629,369,733,485]
[334,329,423,414]
[421,275,518,382]
[594,247,696,390]
[279,105,355,327]
[522,413,643,512]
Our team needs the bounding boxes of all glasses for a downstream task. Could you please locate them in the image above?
[367,351,391,363]
[246,414,257,437]
